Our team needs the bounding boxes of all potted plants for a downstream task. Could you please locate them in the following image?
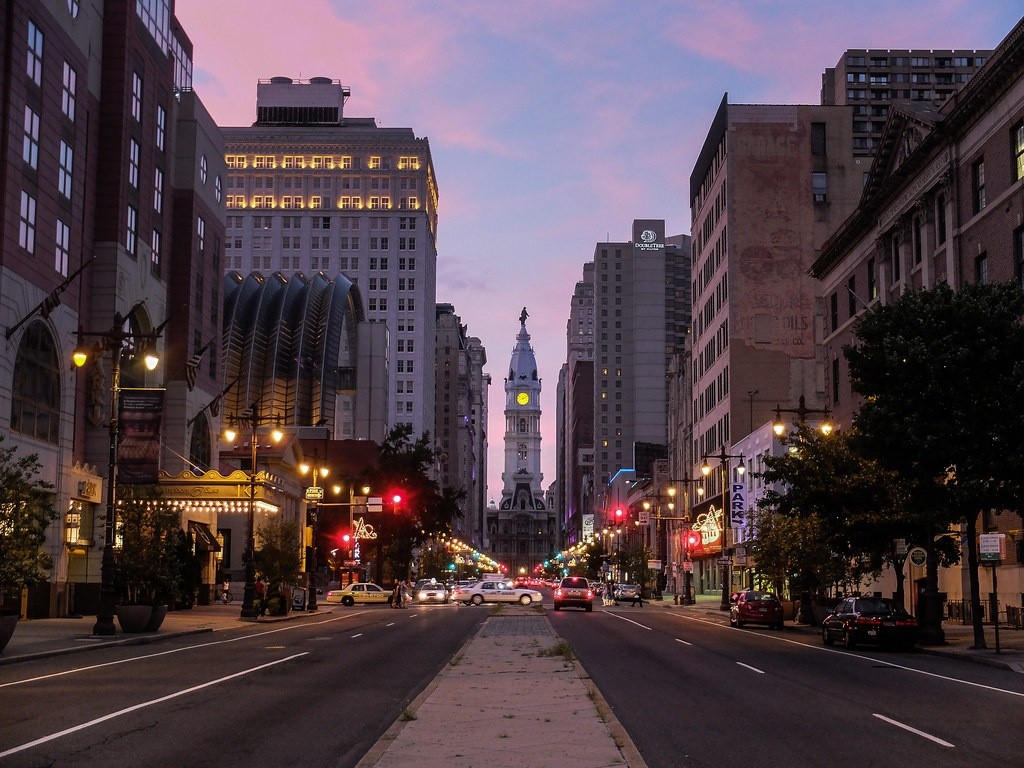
[111,480,193,634]
[0,432,61,651]
[241,517,303,619]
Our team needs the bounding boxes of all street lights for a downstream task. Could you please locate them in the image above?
[663,469,707,605]
[699,444,747,610]
[301,446,329,610]
[332,469,372,584]
[770,390,837,438]
[71,313,166,635]
[643,486,678,603]
[223,401,286,616]
[561,519,621,583]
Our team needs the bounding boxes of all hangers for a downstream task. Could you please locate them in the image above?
[948,600,959,603]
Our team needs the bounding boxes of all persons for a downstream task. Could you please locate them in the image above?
[255,577,268,619]
[600,579,620,607]
[630,580,643,607]
[222,578,230,604]
[391,579,409,609]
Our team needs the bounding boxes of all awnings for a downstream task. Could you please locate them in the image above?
[190,523,221,552]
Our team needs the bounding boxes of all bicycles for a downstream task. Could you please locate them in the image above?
[215,588,233,603]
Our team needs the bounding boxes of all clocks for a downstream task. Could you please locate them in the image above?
[517,392,529,405]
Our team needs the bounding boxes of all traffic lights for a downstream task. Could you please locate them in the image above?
[392,492,403,515]
[689,535,695,549]
[615,508,623,525]
[343,532,351,550]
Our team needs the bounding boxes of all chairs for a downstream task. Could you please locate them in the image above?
[437,586,442,590]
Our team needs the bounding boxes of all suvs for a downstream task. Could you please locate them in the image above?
[553,576,594,612]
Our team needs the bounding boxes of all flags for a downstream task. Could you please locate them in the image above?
[241,402,258,432]
[210,387,230,417]
[92,320,124,351]
[38,278,74,320]
[184,346,208,392]
[136,325,163,355]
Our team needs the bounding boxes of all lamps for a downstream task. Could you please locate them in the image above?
[112,512,124,554]
[63,505,81,552]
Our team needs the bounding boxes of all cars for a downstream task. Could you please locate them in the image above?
[729,591,786,630]
[452,580,544,607]
[418,578,449,605]
[444,571,561,592]
[327,583,412,607]
[413,578,430,600]
[589,582,639,600]
[821,595,920,652]
